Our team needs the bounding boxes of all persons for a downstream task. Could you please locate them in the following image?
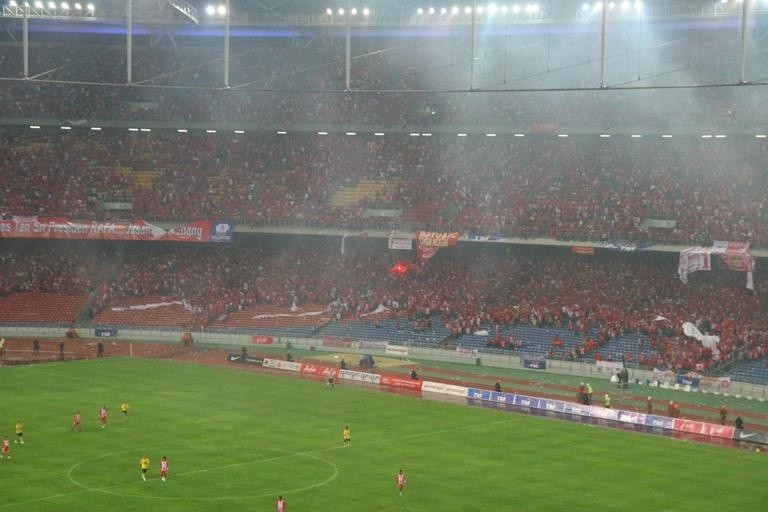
[342,425,352,446]
[139,453,149,483]
[0,435,15,461]
[159,456,168,481]
[273,495,288,512]
[396,469,407,492]
[13,418,27,446]
